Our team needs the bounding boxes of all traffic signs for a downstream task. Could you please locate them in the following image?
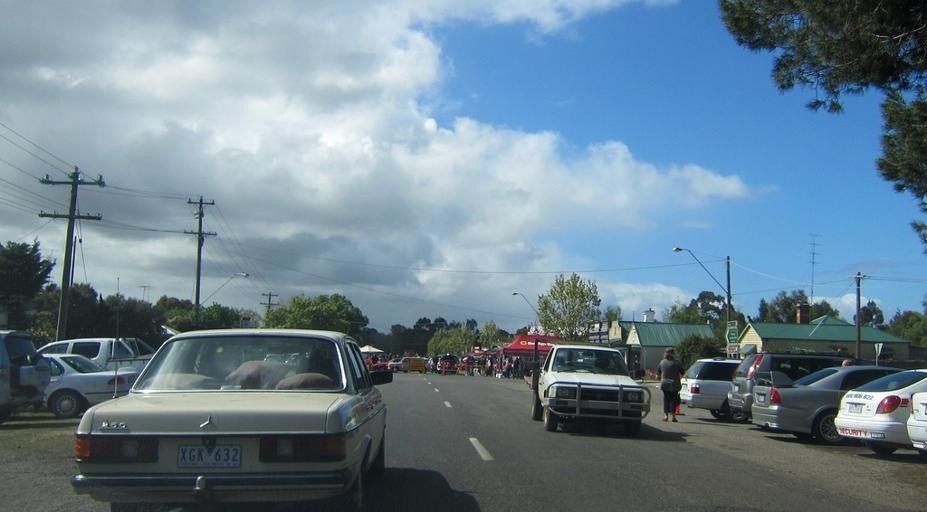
[726,328,739,343]
[727,344,738,354]
[727,321,737,328]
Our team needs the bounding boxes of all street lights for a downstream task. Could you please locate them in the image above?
[512,292,541,318]
[673,247,731,319]
[190,270,249,327]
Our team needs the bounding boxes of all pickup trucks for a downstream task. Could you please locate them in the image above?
[33,337,156,377]
[523,344,649,432]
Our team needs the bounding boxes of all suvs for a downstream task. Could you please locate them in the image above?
[0,330,51,423]
[727,352,871,422]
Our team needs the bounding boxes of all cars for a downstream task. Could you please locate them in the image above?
[750,365,910,444]
[69,328,394,510]
[36,353,140,417]
[833,368,927,459]
[905,391,926,458]
[389,353,496,374]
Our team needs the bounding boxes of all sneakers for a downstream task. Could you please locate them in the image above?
[661,418,668,422]
[672,418,678,422]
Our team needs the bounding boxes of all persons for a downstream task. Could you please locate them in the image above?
[366,350,520,379]
[655,347,686,422]
[842,359,852,366]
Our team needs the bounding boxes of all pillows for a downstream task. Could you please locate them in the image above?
[275,372,334,389]
[225,360,293,389]
[145,373,215,390]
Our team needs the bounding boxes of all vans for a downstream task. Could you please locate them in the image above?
[678,358,749,422]
